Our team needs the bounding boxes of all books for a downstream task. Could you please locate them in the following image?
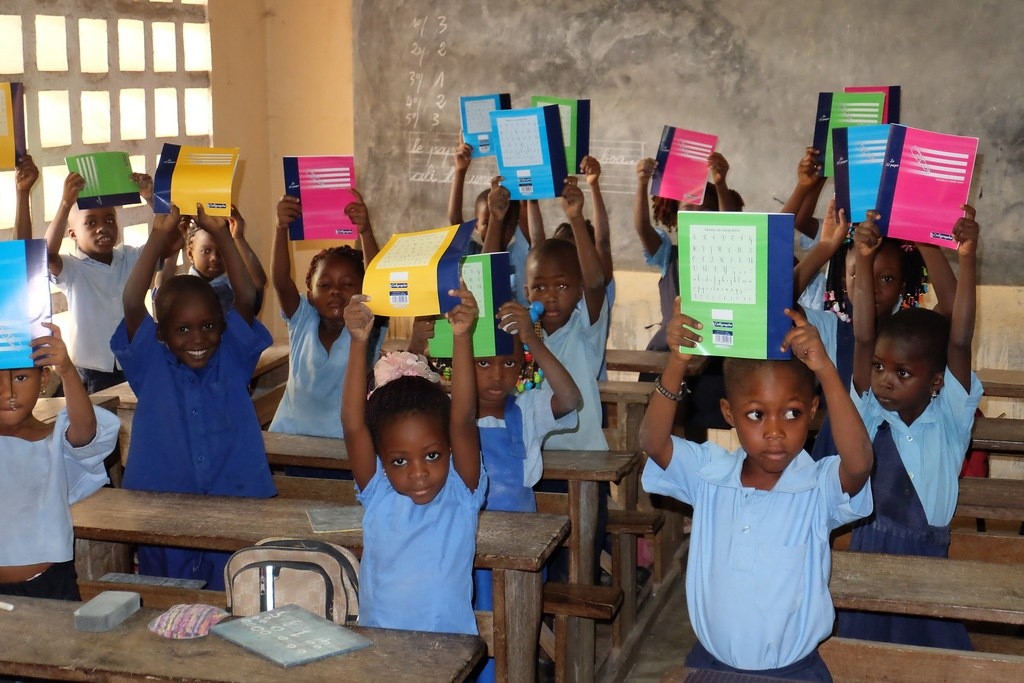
[209,603,374,669]
[0,82,980,366]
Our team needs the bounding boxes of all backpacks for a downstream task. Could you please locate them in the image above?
[224,538,360,624]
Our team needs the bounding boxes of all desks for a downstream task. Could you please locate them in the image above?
[263,430,643,683]
[0,595,489,683]
[606,349,672,375]
[973,369,1024,398]
[817,549,1024,683]
[598,380,685,582]
[31,394,129,592]
[88,343,292,467]
[969,418,1024,456]
[947,477,1024,657]
[67,487,571,683]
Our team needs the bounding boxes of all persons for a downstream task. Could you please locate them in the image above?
[340,277,492,636]
[831,204,985,650]
[0,321,121,604]
[637,295,874,683]
[410,300,582,683]
[13,150,989,594]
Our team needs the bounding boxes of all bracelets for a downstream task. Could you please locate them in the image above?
[654,374,687,402]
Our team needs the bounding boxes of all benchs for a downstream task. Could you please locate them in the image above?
[606,510,666,649]
[542,580,624,683]
